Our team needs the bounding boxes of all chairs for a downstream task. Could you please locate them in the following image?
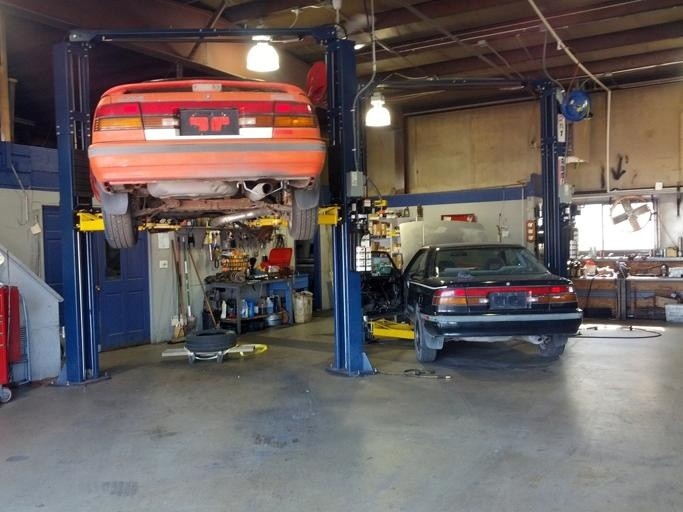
[437,257,505,270]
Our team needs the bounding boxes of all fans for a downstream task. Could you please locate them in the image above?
[608,195,654,232]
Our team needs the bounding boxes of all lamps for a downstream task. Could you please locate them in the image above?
[244,0,437,128]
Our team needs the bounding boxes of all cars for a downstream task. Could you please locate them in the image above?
[361,243,583,362]
[85,76,328,249]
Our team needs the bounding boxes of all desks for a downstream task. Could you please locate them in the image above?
[206,273,308,335]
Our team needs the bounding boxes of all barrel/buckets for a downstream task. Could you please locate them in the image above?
[294,290,313,322]
[265,296,273,313]
[241,299,249,319]
[220,300,227,319]
[258,296,265,314]
